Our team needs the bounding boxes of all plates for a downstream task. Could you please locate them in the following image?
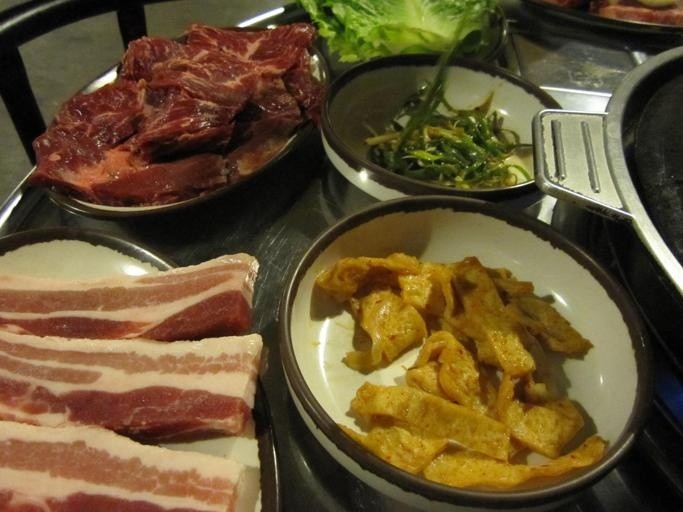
[41,23,332,222]
[1,225,281,511]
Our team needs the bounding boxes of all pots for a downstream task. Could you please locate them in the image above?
[531,42,683,367]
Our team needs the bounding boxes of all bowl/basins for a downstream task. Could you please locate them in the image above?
[276,190,657,510]
[320,51,569,212]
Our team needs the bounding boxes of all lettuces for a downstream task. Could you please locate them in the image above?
[302,0,499,71]
